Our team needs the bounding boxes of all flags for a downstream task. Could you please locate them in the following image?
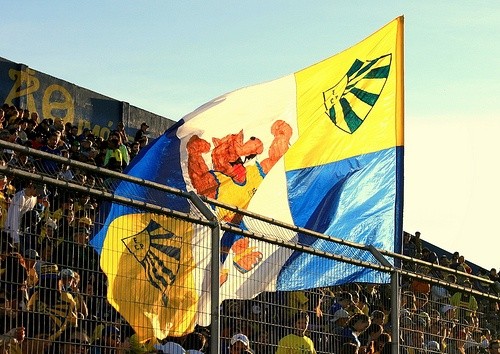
[89,21,398,347]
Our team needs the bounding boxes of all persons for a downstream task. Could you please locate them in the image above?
[0,98,500,354]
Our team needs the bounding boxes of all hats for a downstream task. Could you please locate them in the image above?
[329,310,349,321]
[276,231,500,354]
[155,342,185,354]
[0,104,152,329]
[101,326,120,335]
[230,334,250,350]
[251,306,262,315]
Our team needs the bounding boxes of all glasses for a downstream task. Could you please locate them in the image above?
[66,339,90,349]
[108,335,119,340]
